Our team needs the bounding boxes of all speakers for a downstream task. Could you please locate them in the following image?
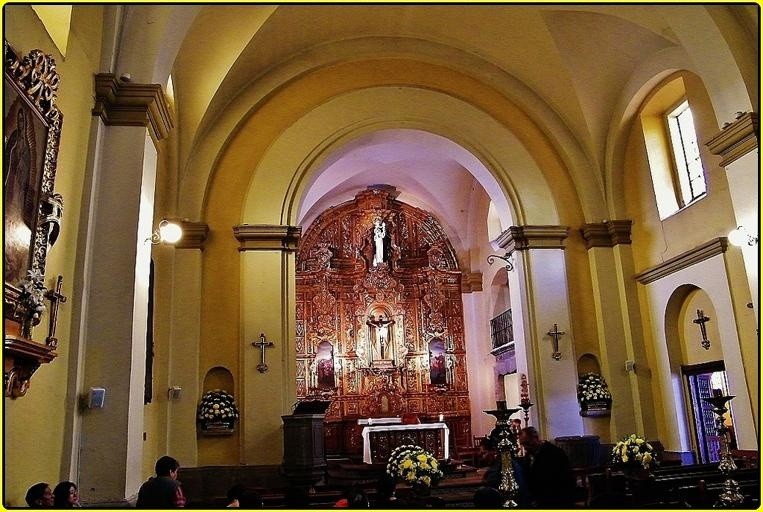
[171,386,181,400]
[87,387,106,409]
[625,360,633,372]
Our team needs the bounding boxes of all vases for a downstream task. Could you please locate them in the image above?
[205,422,229,431]
[586,402,607,410]
[414,484,431,495]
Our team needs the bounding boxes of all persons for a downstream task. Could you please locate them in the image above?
[53,481,81,507]
[25,482,54,506]
[474,486,503,508]
[136,455,187,507]
[479,440,531,508]
[520,426,577,509]
[373,222,386,263]
[368,320,393,352]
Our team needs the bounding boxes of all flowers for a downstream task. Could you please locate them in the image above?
[197,388,239,421]
[385,442,444,482]
[610,432,653,466]
[577,370,611,402]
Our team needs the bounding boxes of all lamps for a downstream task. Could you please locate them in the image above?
[144,217,182,247]
[728,225,757,248]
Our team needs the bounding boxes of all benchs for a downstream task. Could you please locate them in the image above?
[580,461,759,506]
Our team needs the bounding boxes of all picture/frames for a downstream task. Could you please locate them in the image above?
[2,36,77,399]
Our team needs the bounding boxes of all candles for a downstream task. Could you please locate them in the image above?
[713,389,722,397]
[438,414,443,421]
[368,417,372,425]
[496,400,505,409]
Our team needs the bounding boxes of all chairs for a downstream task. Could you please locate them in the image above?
[472,434,488,448]
